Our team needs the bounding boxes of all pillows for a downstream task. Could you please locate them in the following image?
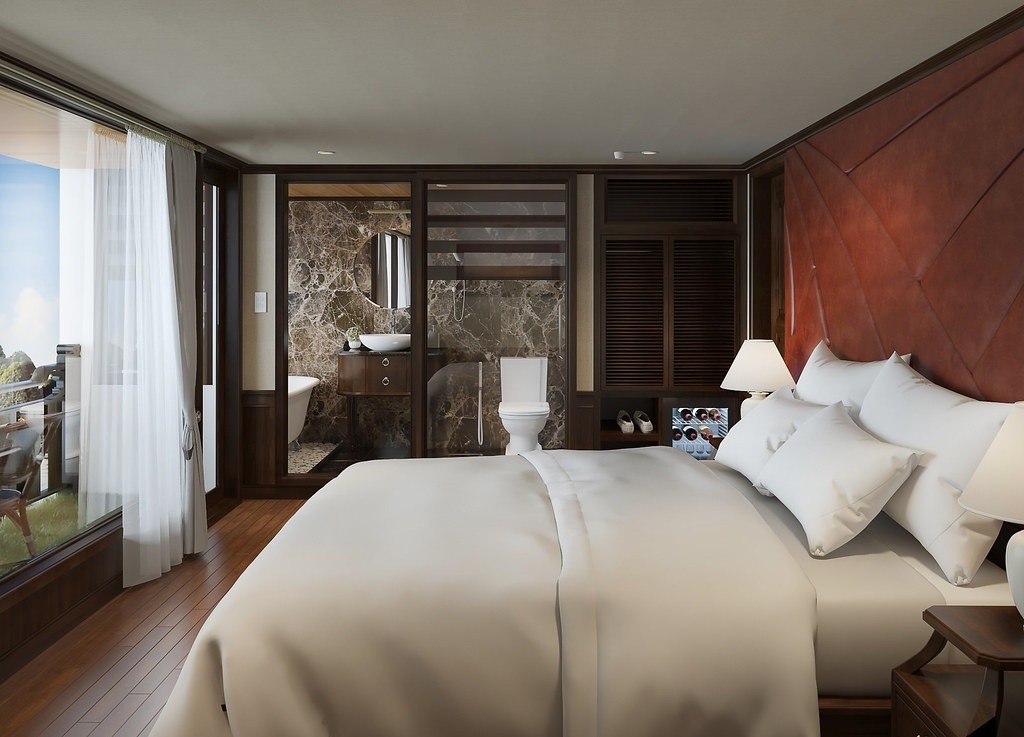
[714,341,1024,587]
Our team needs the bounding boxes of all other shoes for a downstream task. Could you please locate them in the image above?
[634,411,653,434]
[617,410,634,434]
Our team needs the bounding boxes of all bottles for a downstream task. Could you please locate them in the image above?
[682,425,698,441]
[697,425,713,441]
[671,425,683,440]
[692,408,708,421]
[677,408,693,421]
[706,408,721,422]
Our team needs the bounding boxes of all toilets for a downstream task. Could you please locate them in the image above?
[498,356,551,456]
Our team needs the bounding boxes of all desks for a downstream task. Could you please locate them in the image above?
[336,341,442,449]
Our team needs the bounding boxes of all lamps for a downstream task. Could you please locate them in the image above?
[957,401,1024,627]
[720,340,797,420]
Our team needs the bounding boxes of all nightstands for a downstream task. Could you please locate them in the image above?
[890,606,1024,737]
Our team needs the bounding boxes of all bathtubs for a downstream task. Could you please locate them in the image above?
[288,376,320,451]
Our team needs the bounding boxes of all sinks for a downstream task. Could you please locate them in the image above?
[358,334,411,352]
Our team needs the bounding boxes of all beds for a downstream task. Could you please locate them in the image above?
[147,341,1024,737]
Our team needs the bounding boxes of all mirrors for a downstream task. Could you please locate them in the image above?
[353,229,435,310]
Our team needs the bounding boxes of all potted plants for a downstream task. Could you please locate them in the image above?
[344,327,365,348]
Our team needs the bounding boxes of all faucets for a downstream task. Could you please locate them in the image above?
[387,314,396,334]
